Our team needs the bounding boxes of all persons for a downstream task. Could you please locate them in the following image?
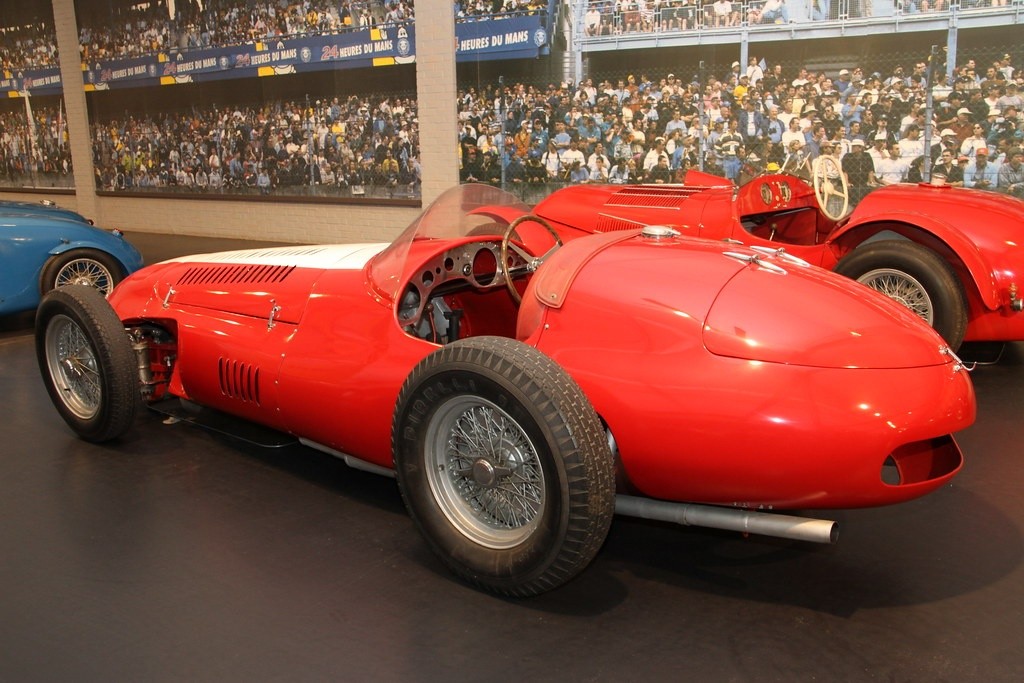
[0,0,1024,207]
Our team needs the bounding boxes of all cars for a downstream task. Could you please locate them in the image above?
[0,197,145,329]
[464,140,1023,360]
[32,174,978,599]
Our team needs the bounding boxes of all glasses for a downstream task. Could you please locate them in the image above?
[973,126,981,130]
[1002,57,1011,62]
[896,82,904,85]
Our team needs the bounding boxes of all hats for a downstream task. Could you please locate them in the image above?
[762,68,773,75]
[844,91,856,100]
[976,148,988,156]
[826,90,839,97]
[865,78,874,84]
[647,97,655,100]
[881,95,891,101]
[668,73,674,79]
[947,91,966,101]
[838,70,849,75]
[802,105,818,114]
[819,140,835,148]
[986,83,1001,91]
[1005,80,1016,87]
[890,78,905,85]
[988,110,1003,117]
[520,120,529,127]
[954,77,966,84]
[739,74,748,80]
[731,61,740,69]
[850,138,865,147]
[939,128,957,138]
[721,100,730,107]
[852,76,861,83]
[681,134,691,142]
[1006,105,1021,112]
[870,72,880,80]
[876,114,889,120]
[874,134,886,141]
[582,113,590,118]
[957,156,969,163]
[893,64,903,71]
[957,108,974,117]
[748,153,761,162]
[715,117,727,122]
[533,136,541,141]
[767,162,780,172]
